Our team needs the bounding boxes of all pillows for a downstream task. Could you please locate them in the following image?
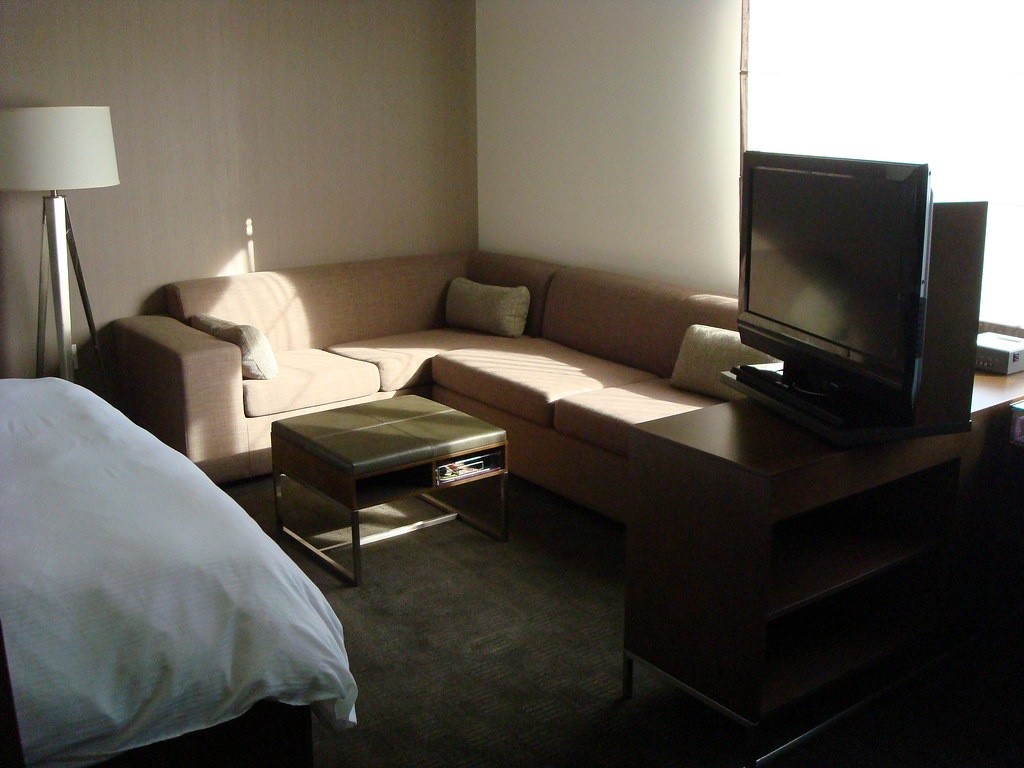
[445,276,531,337]
[188,313,276,379]
[669,323,783,402]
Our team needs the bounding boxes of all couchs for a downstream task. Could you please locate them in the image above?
[112,249,783,527]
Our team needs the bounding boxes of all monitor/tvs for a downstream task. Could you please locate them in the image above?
[730,150,936,429]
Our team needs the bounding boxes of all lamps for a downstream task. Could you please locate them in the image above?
[1,103,120,409]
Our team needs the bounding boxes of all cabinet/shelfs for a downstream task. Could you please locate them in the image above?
[622,370,1024,768]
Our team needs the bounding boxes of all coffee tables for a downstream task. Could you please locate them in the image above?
[271,393,508,590]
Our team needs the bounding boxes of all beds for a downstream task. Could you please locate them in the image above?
[0,377,362,768]
